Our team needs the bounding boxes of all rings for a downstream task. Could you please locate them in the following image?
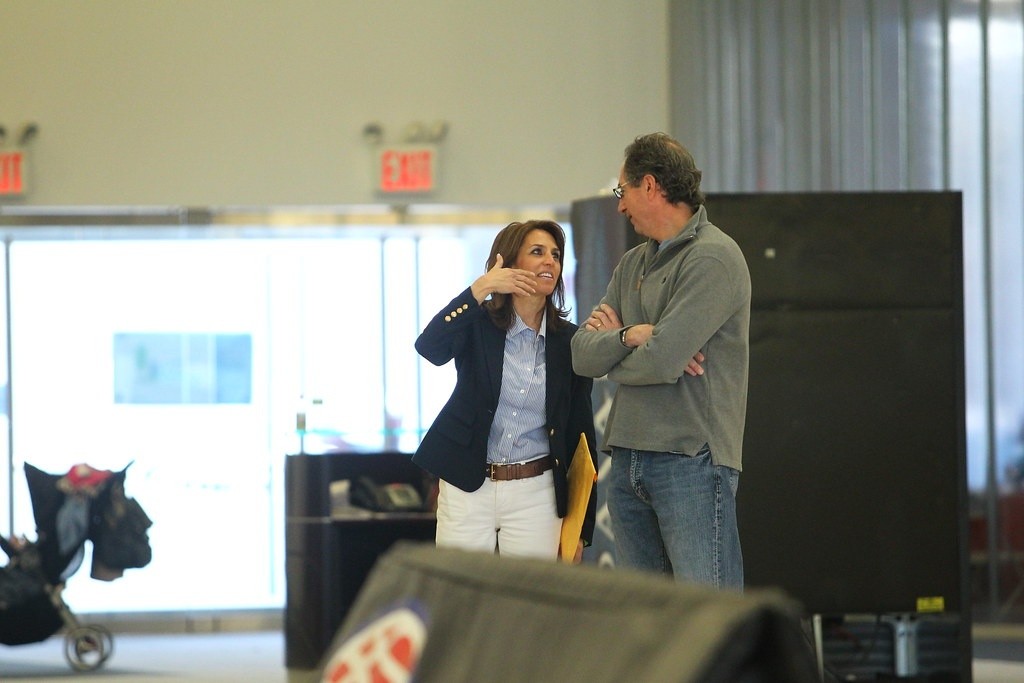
[596,323,602,329]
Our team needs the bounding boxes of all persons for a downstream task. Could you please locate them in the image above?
[569,132,751,596]
[415,220,598,566]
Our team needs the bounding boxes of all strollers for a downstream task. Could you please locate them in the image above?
[0,460,136,676]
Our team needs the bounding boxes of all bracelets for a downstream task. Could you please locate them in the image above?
[621,329,626,343]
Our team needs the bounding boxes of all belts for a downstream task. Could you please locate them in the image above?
[484,455,552,482]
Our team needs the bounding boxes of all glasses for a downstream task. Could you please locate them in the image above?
[613,178,636,199]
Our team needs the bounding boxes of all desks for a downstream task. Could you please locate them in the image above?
[284,451,437,664]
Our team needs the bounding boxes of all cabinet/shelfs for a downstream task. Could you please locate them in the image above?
[570,188,970,621]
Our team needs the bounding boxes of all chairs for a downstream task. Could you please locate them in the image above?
[329,542,821,683]
